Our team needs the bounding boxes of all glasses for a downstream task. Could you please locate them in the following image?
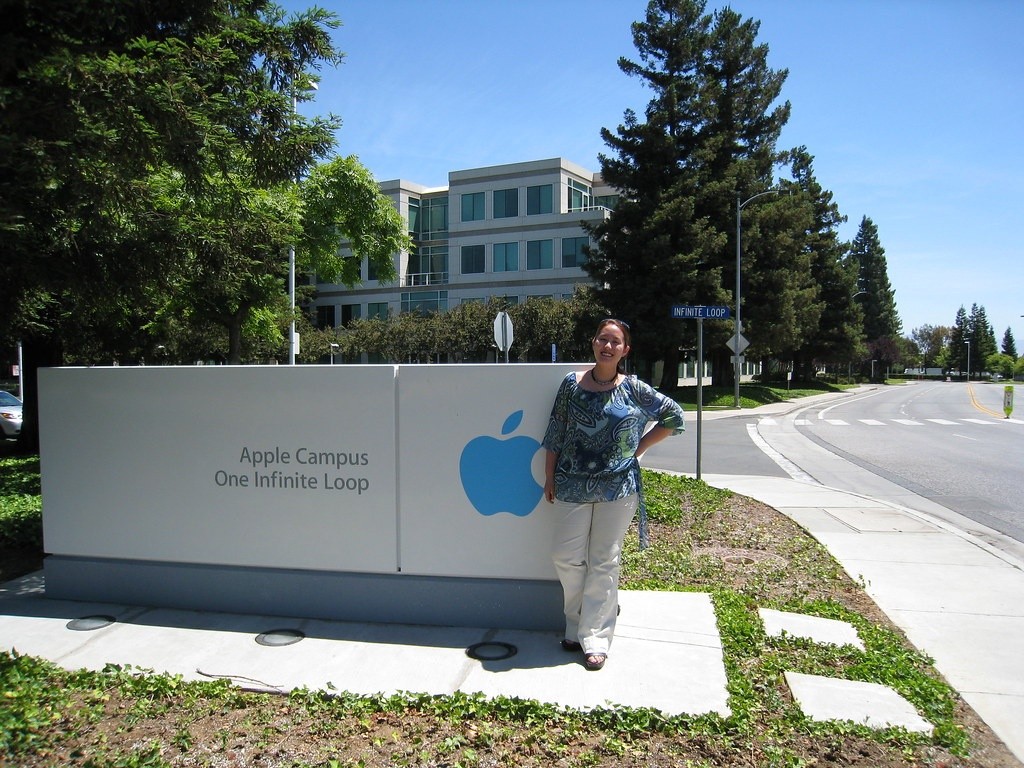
[599,318,630,332]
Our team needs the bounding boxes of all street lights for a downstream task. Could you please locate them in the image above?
[735,189,780,409]
[964,341,970,382]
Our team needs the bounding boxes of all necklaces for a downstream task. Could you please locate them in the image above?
[591,368,619,385]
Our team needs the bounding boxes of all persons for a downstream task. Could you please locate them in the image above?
[536,319,685,671]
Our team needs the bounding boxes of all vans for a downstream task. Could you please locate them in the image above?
[0,389,24,441]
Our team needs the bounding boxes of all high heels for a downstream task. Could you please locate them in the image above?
[562,639,580,652]
[584,652,607,671]
